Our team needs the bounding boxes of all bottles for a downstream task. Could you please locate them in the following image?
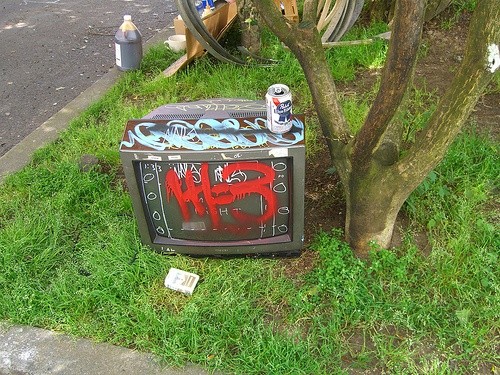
[114,15,143,72]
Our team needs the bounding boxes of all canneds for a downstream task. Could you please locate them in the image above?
[195,0,204,16]
[265,82,294,135]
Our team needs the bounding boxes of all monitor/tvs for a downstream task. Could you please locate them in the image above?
[120,99,306,257]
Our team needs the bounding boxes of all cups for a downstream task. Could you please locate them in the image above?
[163,35,186,54]
